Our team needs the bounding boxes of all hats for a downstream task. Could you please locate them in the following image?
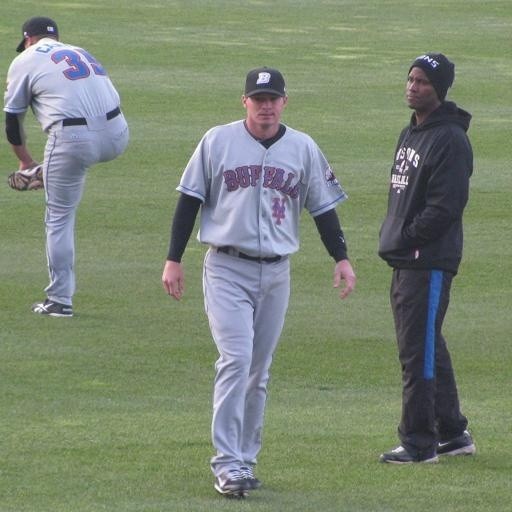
[16,17,58,52]
[245,67,286,96]
[408,53,454,101]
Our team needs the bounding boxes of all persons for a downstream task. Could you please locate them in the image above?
[0,15,132,317]
[161,64,356,496]
[377,53,477,466]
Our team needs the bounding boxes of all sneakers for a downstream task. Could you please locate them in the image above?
[380,446,439,464]
[8,163,45,191]
[214,466,261,494]
[31,299,73,317]
[436,431,475,455]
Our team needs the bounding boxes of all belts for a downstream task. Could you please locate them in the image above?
[63,107,120,126]
[217,246,282,262]
